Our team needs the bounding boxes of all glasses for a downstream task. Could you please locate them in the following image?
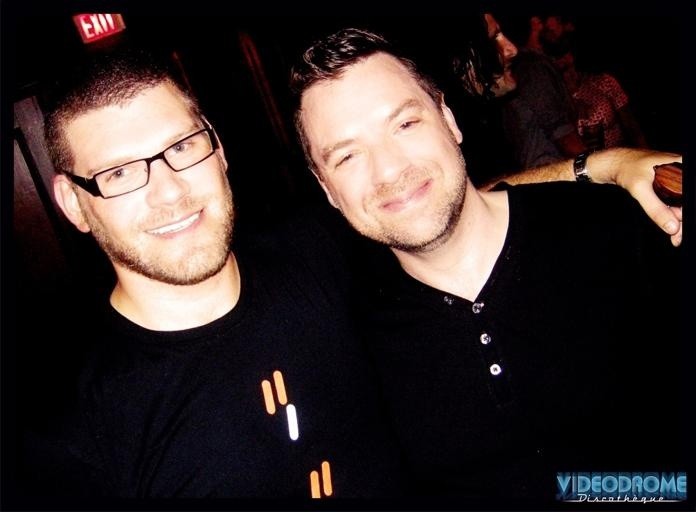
[61,120,217,199]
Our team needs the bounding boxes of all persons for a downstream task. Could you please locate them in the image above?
[285,28,683,497]
[17,59,683,498]
[430,12,646,170]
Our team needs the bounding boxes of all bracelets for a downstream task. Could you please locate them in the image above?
[574,150,592,183]
